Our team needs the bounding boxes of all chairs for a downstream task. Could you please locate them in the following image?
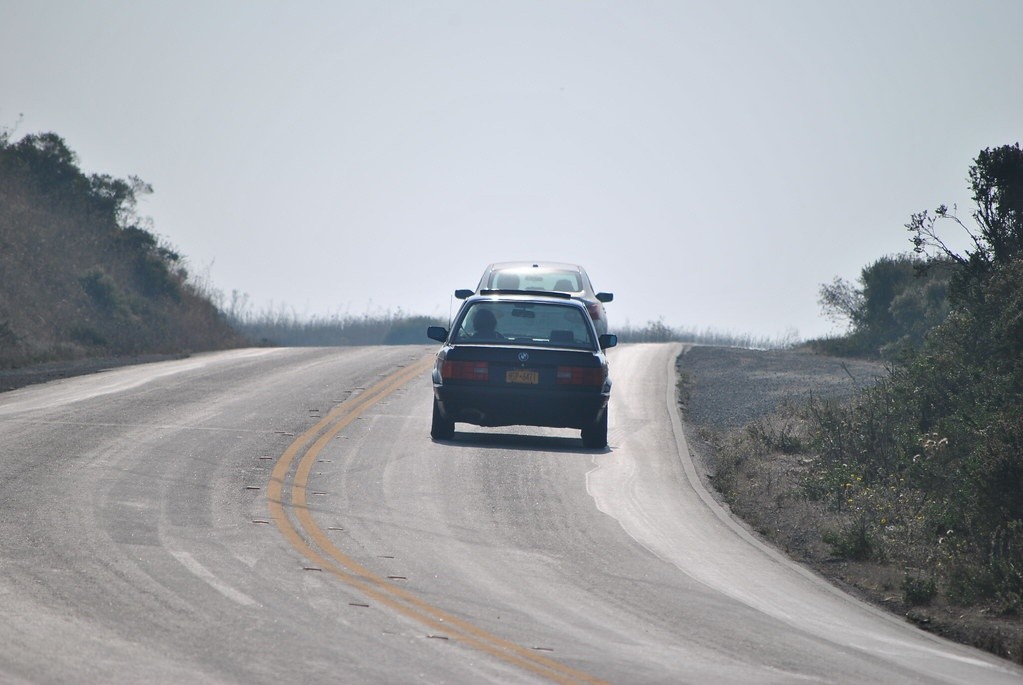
[550,330,574,342]
[552,279,575,292]
[497,275,519,290]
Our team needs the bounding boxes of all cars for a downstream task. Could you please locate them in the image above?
[453,261,614,357]
[426,296,612,449]
